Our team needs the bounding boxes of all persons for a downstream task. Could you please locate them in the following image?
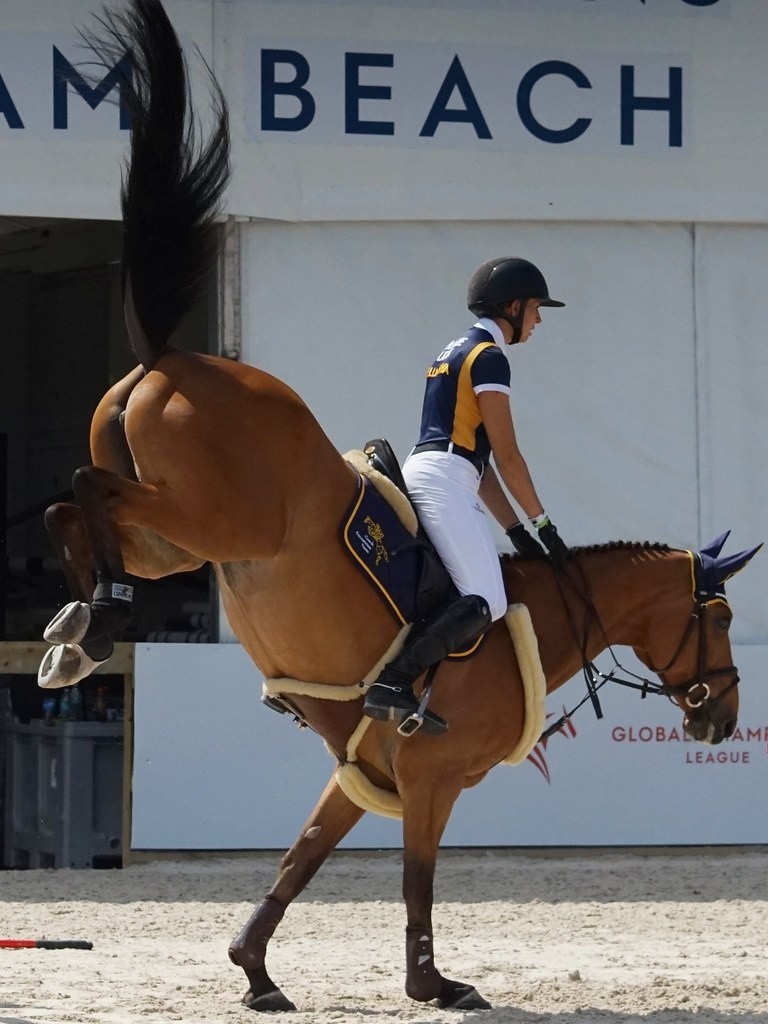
[362,254,578,736]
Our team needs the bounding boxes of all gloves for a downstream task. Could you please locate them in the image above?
[529,511,568,562]
[505,523,544,557]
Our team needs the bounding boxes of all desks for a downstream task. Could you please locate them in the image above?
[3,716,123,870]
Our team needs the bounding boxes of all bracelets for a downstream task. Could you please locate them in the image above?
[530,508,549,529]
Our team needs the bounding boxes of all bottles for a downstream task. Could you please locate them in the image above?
[59,689,70,717]
[70,682,83,720]
[95,688,107,722]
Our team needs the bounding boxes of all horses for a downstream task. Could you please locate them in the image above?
[37,0,764,1012]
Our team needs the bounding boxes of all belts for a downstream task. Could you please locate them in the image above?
[412,443,484,475]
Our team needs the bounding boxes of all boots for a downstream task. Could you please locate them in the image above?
[362,594,491,732]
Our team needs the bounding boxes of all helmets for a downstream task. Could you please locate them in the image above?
[467,257,565,307]
